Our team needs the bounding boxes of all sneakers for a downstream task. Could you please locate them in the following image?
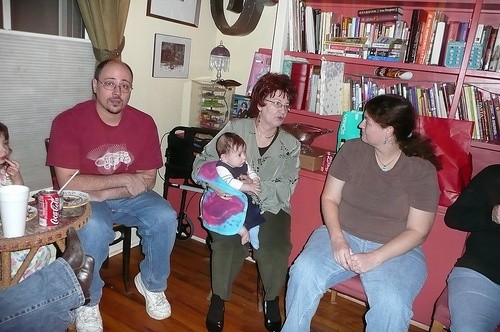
[73,303,104,332]
[133,272,172,321]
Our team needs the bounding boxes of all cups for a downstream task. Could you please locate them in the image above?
[59,191,65,218]
[0,184,29,238]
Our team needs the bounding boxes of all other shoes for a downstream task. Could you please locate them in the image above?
[262,295,282,332]
[205,292,226,332]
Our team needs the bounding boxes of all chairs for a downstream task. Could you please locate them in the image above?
[45,138,131,292]
[330,274,367,305]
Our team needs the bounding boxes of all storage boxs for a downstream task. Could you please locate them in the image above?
[299,153,322,172]
[247,49,272,95]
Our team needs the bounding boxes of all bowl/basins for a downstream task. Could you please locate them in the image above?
[281,123,334,154]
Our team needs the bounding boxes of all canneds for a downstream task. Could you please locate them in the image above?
[38,190,59,227]
[321,152,334,173]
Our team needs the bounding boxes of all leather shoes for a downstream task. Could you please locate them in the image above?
[59,225,96,307]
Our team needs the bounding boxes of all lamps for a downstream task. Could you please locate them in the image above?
[209,40,230,82]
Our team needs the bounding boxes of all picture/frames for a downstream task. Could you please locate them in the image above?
[145,0,201,28]
[153,33,192,79]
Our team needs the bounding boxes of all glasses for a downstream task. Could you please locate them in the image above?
[97,79,133,93]
[264,98,290,110]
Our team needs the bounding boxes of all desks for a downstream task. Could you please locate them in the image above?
[0,203,92,291]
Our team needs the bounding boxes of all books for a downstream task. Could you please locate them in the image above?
[282,0,500,146]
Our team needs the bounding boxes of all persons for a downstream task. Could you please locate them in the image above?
[236,102,249,118]
[216,132,261,251]
[0,122,57,283]
[280,94,441,332]
[444,163,500,332]
[45,59,177,332]
[192,72,302,332]
[0,226,96,332]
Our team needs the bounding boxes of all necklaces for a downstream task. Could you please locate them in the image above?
[256,125,277,139]
[376,152,400,169]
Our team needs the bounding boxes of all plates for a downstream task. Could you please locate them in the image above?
[29,190,90,208]
[26,205,38,221]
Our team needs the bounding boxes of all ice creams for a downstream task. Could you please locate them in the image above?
[246,161,258,180]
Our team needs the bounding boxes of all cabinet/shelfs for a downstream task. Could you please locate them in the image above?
[270,0,500,153]
[167,147,468,331]
[187,80,234,139]
